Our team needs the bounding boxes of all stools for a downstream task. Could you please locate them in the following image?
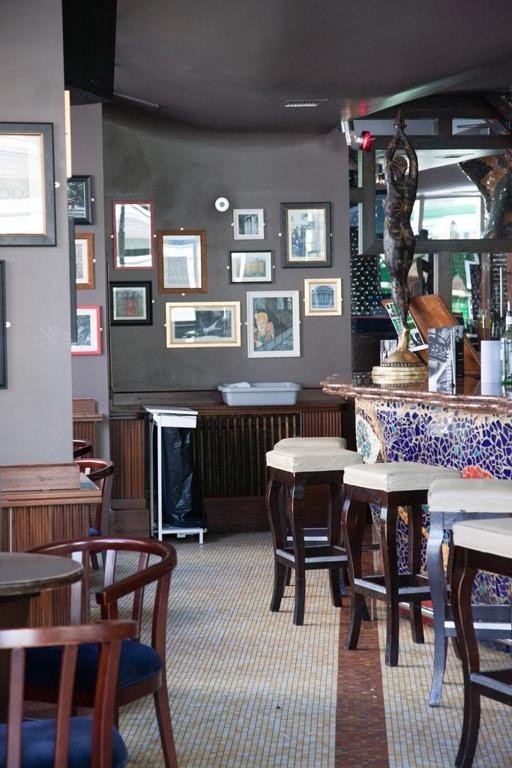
[342,457,511,764]
[266,437,372,628]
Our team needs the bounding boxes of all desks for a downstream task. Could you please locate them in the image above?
[0,551,86,724]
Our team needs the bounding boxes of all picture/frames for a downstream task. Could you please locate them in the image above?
[109,280,152,326]
[233,208,265,240]
[279,200,333,269]
[296,246,297,247]
[75,232,95,289]
[230,250,274,284]
[165,301,242,348]
[72,174,93,225]
[164,238,200,287]
[0,123,56,246]
[246,290,301,359]
[304,278,343,317]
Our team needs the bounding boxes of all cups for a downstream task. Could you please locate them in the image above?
[481,383,500,396]
[480,340,500,383]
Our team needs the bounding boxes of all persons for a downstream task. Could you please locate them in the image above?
[380,107,428,361]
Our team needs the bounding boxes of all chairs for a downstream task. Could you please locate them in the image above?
[1,619,138,766]
[18,534,177,767]
[74,457,114,570]
[73,439,92,457]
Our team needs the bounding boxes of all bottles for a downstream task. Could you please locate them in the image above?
[449,221,456,239]
[352,371,373,386]
[476,299,511,339]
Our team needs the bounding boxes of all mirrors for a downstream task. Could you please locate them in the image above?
[156,229,207,293]
[111,198,154,270]
